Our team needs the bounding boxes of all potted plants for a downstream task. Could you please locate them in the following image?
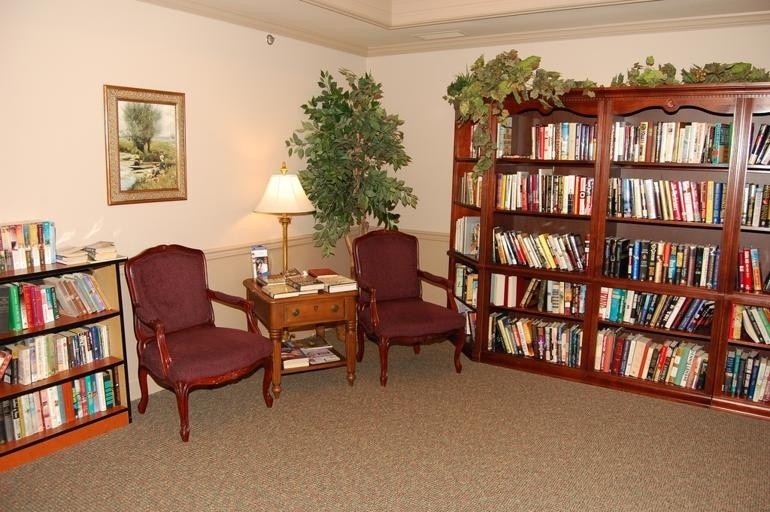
[285,66,418,343]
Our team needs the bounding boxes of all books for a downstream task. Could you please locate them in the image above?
[257,269,359,299]
[741,183,770,227]
[280,337,341,368]
[454,123,482,342]
[488,116,598,366]
[0,222,126,448]
[721,246,770,405]
[749,123,770,165]
[594,120,732,390]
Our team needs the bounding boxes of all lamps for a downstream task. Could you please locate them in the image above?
[254,161,316,280]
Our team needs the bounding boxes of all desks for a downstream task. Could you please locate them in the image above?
[243,278,359,399]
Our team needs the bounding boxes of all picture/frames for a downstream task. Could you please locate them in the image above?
[103,85,187,205]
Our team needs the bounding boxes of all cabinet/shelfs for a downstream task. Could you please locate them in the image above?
[447,82,770,422]
[0,255,128,473]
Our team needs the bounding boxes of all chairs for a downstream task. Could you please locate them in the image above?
[352,230,466,387]
[125,244,273,442]
